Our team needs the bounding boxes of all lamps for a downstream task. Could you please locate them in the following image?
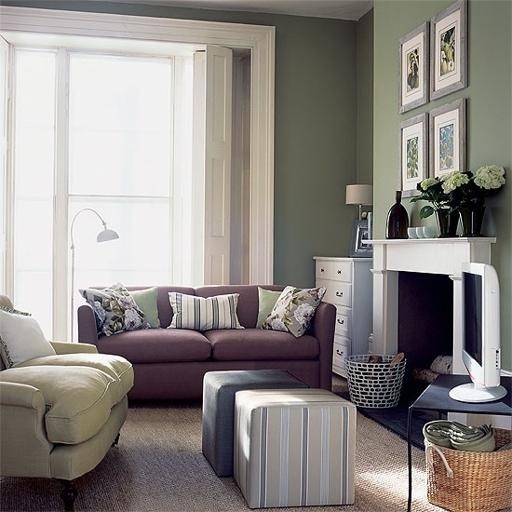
[70,208,120,342]
[346,184,372,220]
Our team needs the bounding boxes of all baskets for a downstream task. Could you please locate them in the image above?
[344,354,407,408]
[424,427,512,512]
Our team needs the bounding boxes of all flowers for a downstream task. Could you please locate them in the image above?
[409,163,509,220]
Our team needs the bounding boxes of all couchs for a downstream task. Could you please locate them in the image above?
[78,285,337,402]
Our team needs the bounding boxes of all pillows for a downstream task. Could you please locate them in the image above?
[127,287,161,329]
[79,282,152,337]
[0,305,57,369]
[261,285,327,338]
[166,291,245,330]
[256,286,283,329]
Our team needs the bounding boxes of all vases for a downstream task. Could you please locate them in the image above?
[459,202,485,237]
[437,206,460,238]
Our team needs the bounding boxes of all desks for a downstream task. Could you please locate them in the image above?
[406,372,512,512]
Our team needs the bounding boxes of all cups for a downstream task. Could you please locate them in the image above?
[422,226,435,238]
[407,227,417,239]
[415,227,423,239]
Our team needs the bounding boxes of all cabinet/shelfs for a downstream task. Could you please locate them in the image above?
[313,256,373,378]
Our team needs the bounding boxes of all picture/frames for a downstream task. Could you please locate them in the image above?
[397,21,429,115]
[428,97,467,179]
[429,1,468,102]
[349,219,373,258]
[397,112,428,199]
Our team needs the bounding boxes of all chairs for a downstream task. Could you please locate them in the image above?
[0,293,135,512]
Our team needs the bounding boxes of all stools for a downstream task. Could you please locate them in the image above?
[201,368,310,477]
[234,387,357,509]
